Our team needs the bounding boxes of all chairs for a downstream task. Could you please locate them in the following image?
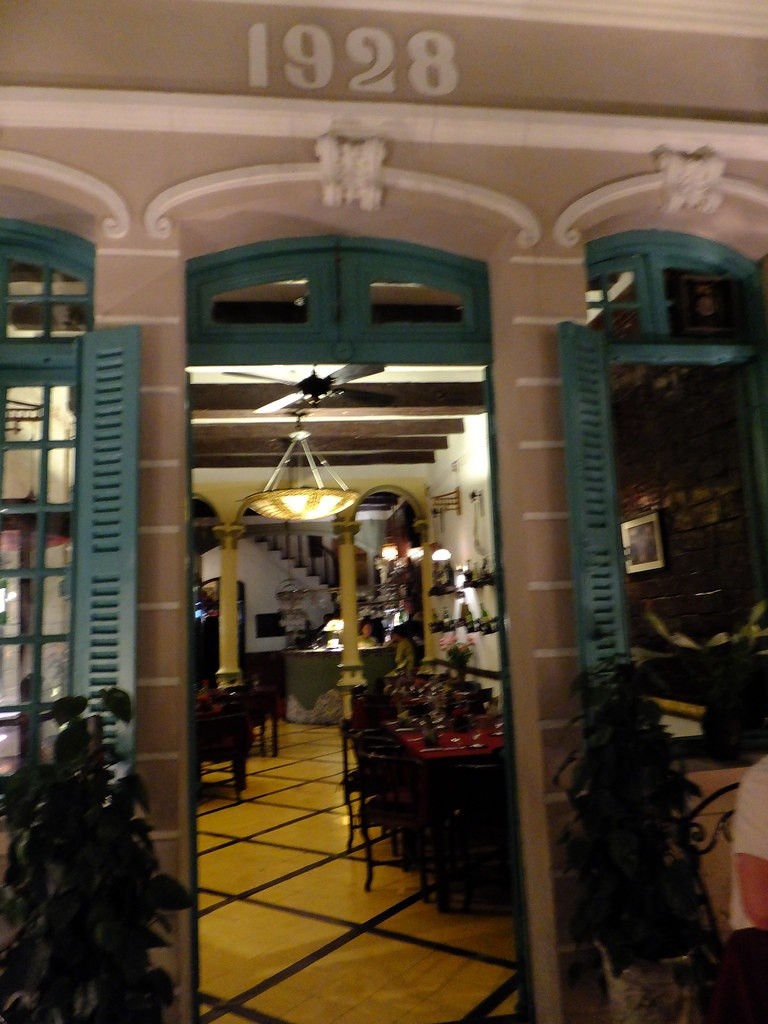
[189,686,279,791]
[339,674,520,914]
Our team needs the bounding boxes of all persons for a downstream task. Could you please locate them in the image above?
[729,753,768,1024]
[356,623,380,647]
[385,631,414,677]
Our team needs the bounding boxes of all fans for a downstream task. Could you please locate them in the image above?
[222,363,402,414]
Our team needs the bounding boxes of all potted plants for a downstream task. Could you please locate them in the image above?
[551,655,724,1024]
[671,610,759,761]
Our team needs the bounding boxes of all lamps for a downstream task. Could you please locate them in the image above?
[242,411,360,522]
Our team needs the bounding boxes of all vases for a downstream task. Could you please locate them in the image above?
[619,508,670,578]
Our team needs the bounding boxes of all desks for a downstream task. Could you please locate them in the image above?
[381,717,502,905]
